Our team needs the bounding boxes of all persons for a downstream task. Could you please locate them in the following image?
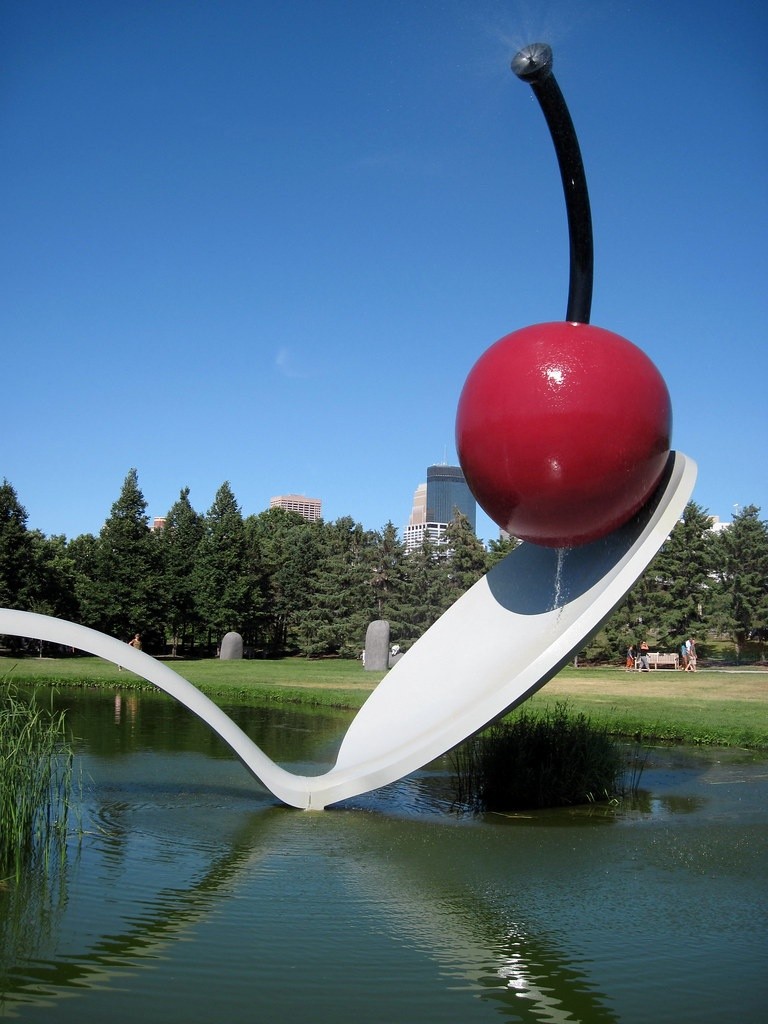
[639,640,651,672]
[360,650,365,667]
[626,645,634,672]
[215,638,222,657]
[249,636,255,658]
[679,638,697,672]
[118,634,143,671]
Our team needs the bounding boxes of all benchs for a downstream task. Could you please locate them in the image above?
[634,652,680,670]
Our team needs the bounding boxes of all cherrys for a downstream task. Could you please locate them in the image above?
[454,44,674,552]
